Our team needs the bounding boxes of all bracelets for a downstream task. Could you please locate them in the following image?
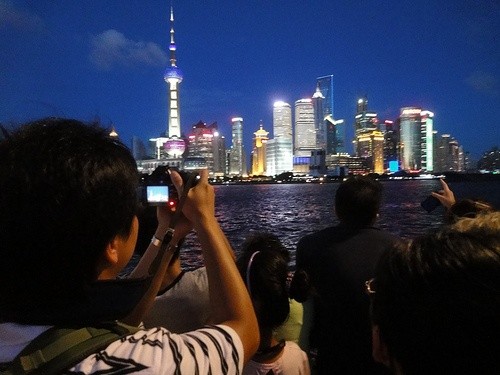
[151,235,161,247]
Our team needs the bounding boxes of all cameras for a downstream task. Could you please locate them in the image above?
[421,191,444,213]
[137,165,200,209]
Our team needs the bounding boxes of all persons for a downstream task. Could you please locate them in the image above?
[0,114,500,374]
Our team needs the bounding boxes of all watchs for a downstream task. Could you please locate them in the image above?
[166,244,178,253]
